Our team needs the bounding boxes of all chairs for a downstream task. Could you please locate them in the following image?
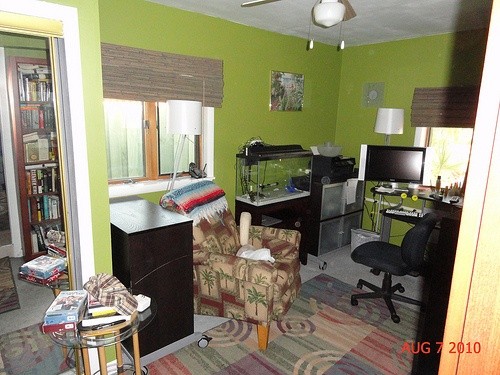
[159,180,302,353]
[351,213,442,323]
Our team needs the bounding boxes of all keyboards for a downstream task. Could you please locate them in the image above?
[386,209,424,218]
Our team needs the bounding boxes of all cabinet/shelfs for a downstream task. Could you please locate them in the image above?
[8,56,67,262]
[291,178,365,221]
[110,220,194,360]
[304,212,362,257]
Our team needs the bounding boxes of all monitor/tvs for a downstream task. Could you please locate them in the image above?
[357,143,432,193]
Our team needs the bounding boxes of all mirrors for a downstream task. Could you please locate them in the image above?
[0,0,101,375]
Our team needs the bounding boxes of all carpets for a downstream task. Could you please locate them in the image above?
[145,273,421,375]
[0,256,21,315]
[0,323,76,375]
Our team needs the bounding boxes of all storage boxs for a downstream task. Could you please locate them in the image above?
[18,254,68,284]
[44,290,88,324]
[234,150,313,207]
[41,323,81,334]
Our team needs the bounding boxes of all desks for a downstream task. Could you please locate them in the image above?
[47,289,158,375]
[16,272,69,297]
[370,185,462,242]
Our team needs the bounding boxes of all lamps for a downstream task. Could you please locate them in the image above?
[166,100,203,191]
[309,0,346,50]
[374,109,404,233]
